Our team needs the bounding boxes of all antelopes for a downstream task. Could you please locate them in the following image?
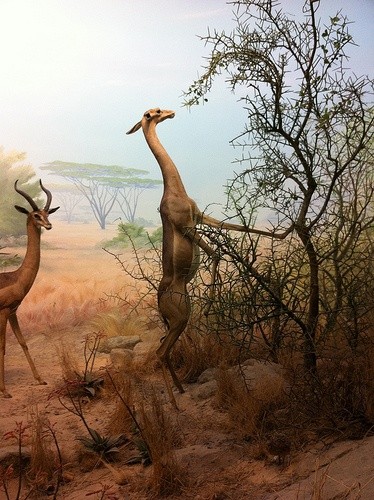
[126,108,295,409]
[0,179,60,398]
